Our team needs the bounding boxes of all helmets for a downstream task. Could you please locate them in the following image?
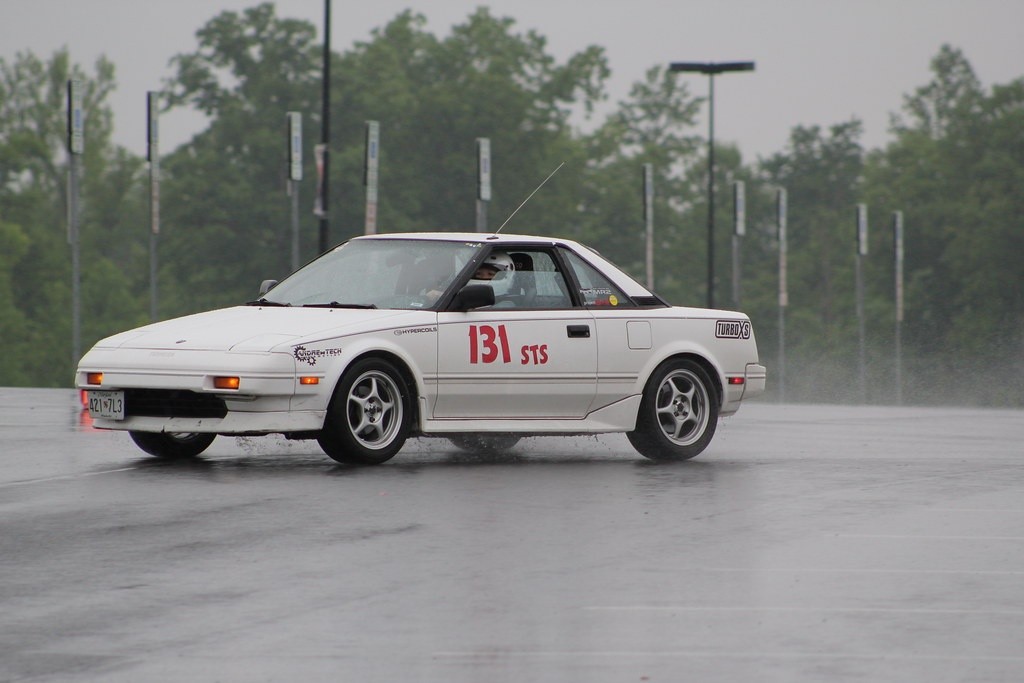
[466,251,515,297]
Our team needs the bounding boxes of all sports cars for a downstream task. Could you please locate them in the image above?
[70,231,767,468]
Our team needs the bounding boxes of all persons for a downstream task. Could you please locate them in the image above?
[454,251,517,311]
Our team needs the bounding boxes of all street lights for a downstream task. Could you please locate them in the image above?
[670,61,757,307]
